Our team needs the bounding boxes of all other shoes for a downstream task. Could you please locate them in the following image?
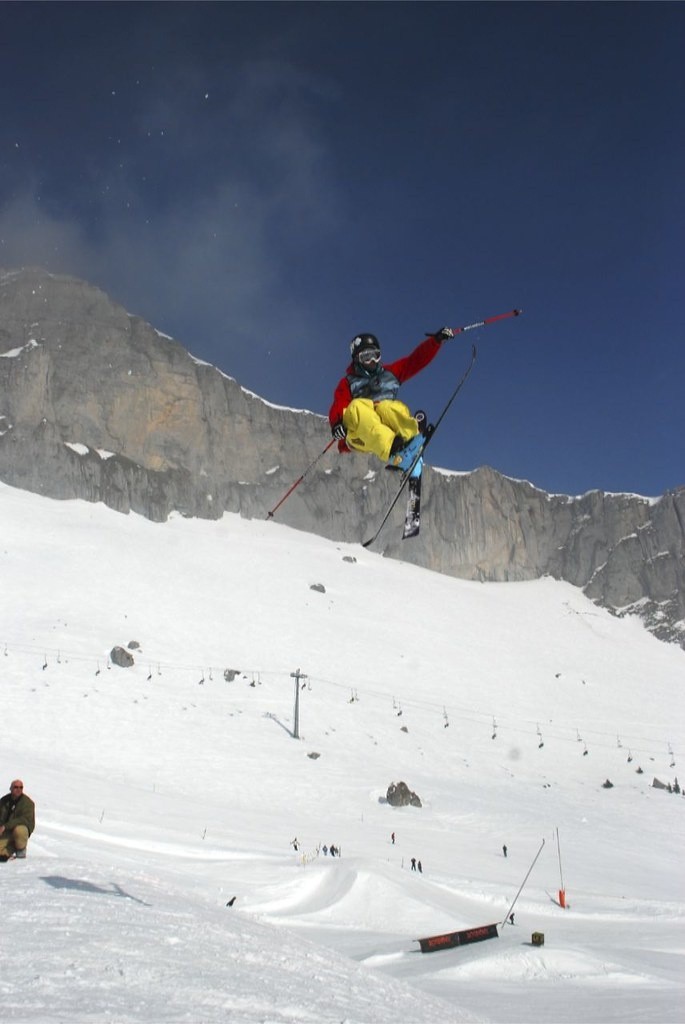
[408,460,423,482]
[16,848,27,859]
[389,432,426,473]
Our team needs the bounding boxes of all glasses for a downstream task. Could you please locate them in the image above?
[13,786,24,790]
[358,349,382,363]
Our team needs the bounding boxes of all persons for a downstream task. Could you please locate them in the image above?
[503,845,507,857]
[418,861,422,872]
[510,913,515,925]
[329,326,456,488]
[291,837,300,851]
[330,845,338,857]
[392,832,395,844]
[323,845,328,856]
[0,779,35,863]
[411,857,416,871]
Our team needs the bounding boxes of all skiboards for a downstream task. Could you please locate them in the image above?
[361,343,479,549]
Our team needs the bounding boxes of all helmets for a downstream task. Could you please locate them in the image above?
[350,333,380,358]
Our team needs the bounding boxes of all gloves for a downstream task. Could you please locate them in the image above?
[332,424,346,441]
[435,327,455,343]
